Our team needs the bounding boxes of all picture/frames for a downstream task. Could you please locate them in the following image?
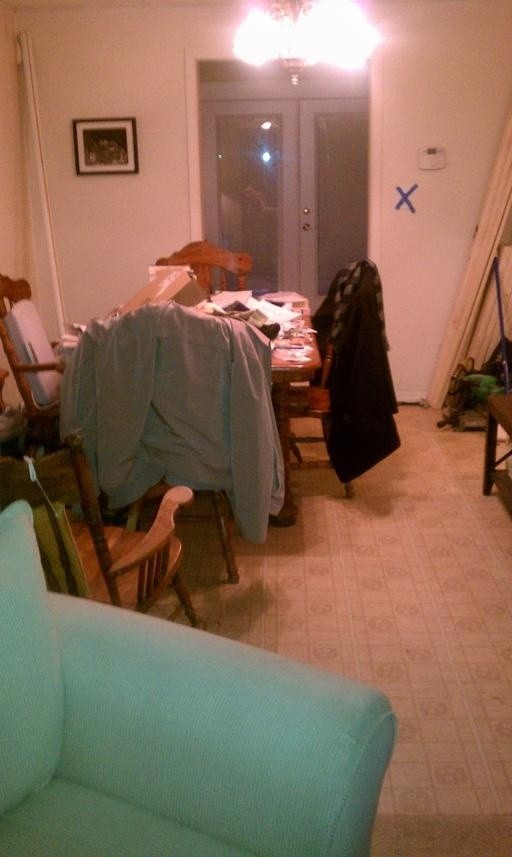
[74,117,140,174]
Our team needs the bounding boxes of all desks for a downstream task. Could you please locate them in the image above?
[185,289,324,525]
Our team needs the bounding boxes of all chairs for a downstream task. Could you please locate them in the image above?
[1,276,62,461]
[276,254,370,499]
[62,302,279,584]
[2,428,202,625]
[155,241,254,307]
